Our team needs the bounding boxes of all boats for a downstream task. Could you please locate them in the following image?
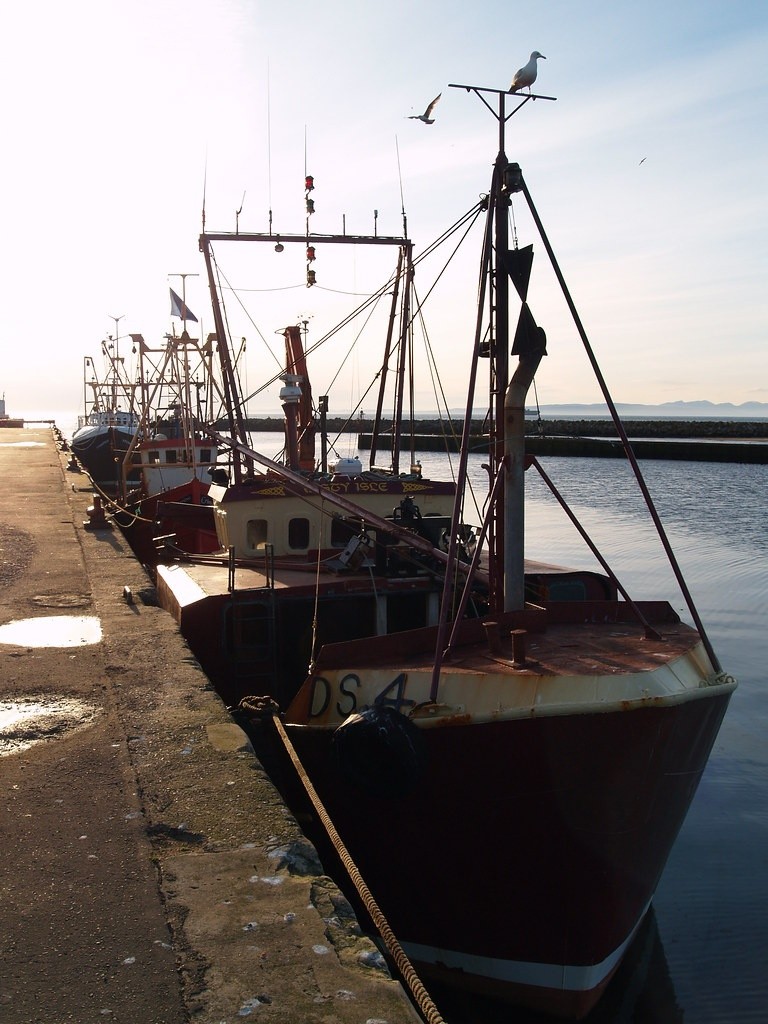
[66,77,744,1024]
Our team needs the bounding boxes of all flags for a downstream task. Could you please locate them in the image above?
[169,288,198,322]
